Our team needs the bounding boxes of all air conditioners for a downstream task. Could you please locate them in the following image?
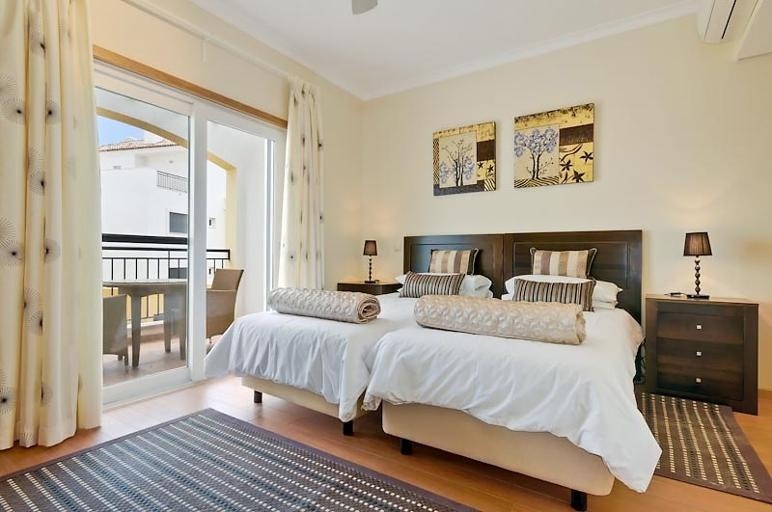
[693,0,758,45]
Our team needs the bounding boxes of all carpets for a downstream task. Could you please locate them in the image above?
[0,406,484,510]
[633,391,772,508]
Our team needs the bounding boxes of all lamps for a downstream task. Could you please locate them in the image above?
[681,230,714,301]
[363,239,378,284]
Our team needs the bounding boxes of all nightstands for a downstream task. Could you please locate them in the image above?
[642,291,761,416]
[336,281,402,296]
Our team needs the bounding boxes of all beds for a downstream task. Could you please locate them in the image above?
[228,231,505,438]
[361,228,645,511]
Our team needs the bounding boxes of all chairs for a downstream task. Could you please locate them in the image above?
[101,293,129,365]
[206,268,246,341]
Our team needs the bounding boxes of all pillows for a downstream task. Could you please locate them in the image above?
[394,245,623,313]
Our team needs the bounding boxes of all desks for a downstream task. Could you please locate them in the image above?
[101,276,188,369]
